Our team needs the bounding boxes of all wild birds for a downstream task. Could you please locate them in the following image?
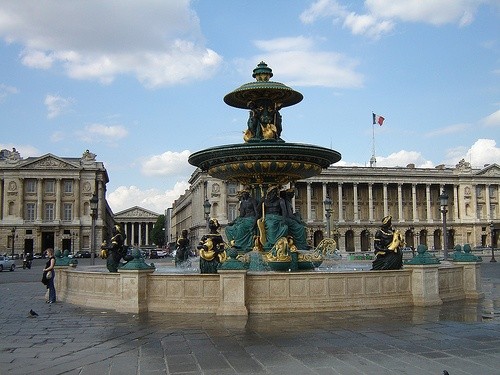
[443,370,449,375]
[29,309,39,317]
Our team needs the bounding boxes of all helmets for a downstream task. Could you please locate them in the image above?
[27,253,29,255]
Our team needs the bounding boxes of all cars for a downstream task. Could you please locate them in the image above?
[139,247,167,259]
[0,256,16,272]
[33,253,42,258]
[60,250,95,259]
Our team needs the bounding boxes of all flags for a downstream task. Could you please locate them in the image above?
[372,112,385,126]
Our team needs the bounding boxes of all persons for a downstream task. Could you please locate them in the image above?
[243,100,286,142]
[25,253,33,267]
[225,185,310,263]
[100,223,127,272]
[197,218,227,274]
[175,229,190,266]
[370,215,407,271]
[41,248,56,304]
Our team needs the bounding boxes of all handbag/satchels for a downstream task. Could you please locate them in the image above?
[46,271,52,279]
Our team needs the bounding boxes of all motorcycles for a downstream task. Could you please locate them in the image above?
[22,258,32,269]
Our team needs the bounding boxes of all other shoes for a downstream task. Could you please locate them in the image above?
[46,300,53,304]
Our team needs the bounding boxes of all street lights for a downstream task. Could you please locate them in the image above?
[88,191,98,266]
[12,228,16,260]
[439,189,449,261]
[323,194,333,238]
[203,198,212,232]
[489,221,497,262]
